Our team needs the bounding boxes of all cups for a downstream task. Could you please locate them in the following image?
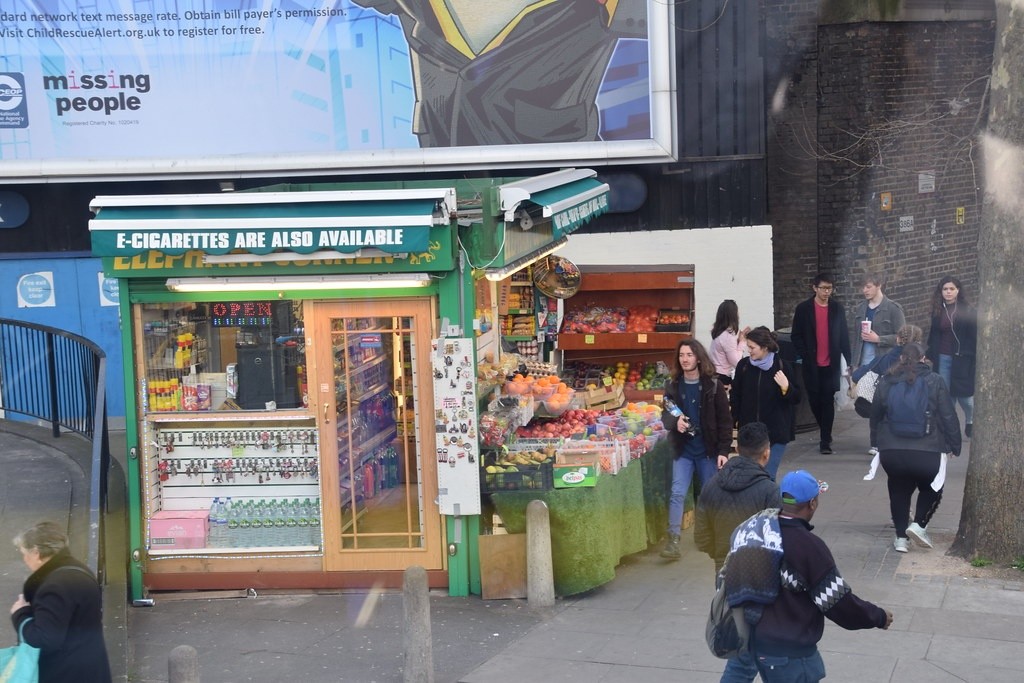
[861,320,871,335]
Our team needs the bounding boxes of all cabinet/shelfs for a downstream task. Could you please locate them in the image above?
[143,305,213,373]
[331,316,403,532]
[500,281,548,342]
[389,336,419,483]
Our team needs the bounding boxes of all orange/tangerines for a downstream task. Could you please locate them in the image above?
[628,403,661,414]
[502,374,574,410]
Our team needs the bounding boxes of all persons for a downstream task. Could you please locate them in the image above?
[661,300,802,558]
[717,470,893,683]
[10,521,112,683]
[924,275,977,437]
[790,273,906,455]
[694,422,782,683]
[851,325,962,553]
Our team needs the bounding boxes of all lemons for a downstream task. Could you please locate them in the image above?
[613,361,629,388]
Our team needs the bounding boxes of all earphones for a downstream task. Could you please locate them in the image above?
[957,290,959,294]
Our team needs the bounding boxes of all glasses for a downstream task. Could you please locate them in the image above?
[817,285,834,292]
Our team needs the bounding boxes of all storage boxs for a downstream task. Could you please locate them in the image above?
[225,363,238,399]
[149,510,210,550]
[182,384,212,411]
[479,383,631,494]
[655,307,691,332]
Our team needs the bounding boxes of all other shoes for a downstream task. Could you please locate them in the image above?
[868,447,878,455]
[820,434,834,454]
[965,420,973,438]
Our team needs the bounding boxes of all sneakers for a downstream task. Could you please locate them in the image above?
[894,537,911,553]
[905,522,933,548]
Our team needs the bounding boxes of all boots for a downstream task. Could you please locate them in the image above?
[659,534,682,559]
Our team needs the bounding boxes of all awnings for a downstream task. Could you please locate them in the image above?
[87,200,437,258]
[529,178,613,240]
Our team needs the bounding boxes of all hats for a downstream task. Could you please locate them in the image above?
[780,471,821,503]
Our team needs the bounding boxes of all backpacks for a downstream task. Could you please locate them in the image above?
[885,371,935,438]
[704,581,752,660]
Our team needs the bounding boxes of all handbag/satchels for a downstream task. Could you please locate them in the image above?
[0,617,42,683]
[856,371,879,403]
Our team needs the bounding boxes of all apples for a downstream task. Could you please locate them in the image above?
[514,409,662,456]
[563,305,691,334]
[628,361,672,390]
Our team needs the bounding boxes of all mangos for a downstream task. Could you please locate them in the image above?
[486,447,555,488]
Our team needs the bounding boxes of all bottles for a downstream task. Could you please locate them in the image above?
[329,319,414,544]
[663,396,693,429]
[207,496,321,548]
[296,365,308,409]
[141,303,209,411]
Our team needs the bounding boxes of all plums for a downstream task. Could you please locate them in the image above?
[561,360,600,388]
